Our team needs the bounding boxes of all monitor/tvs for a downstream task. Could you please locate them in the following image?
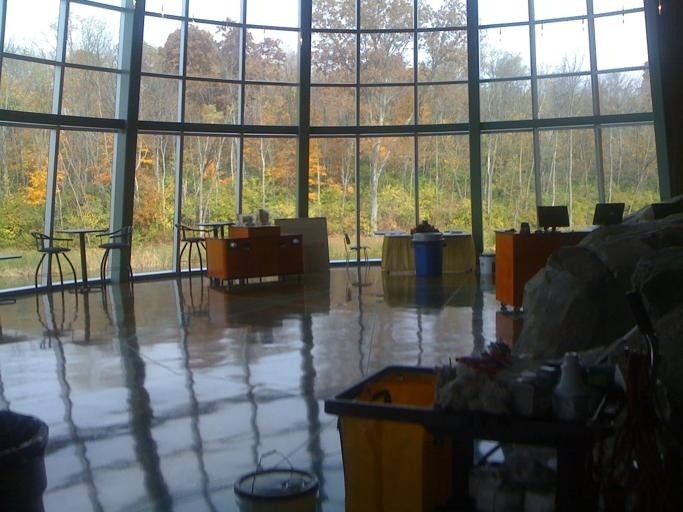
[537,205,570,234]
[592,202,625,225]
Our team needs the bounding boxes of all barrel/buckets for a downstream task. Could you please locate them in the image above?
[479,254,496,274]
[410,232,446,308]
[235,448,322,512]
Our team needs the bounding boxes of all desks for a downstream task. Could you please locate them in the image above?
[55,229,108,292]
[72,291,109,347]
[374,234,474,277]
[195,222,235,238]
[227,226,281,239]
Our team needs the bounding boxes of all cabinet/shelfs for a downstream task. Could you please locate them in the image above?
[206,236,304,287]
[492,230,598,313]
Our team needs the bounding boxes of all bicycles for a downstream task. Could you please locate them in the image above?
[497,287,683,512]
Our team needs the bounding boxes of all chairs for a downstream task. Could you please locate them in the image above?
[33,290,79,350]
[175,223,212,273]
[30,232,78,288]
[101,286,137,339]
[92,226,134,285]
[344,234,371,270]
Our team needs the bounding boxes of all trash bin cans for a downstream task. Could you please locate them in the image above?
[412,231,445,278]
[326,366,460,510]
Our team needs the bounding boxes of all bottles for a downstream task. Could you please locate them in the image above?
[551,352,591,425]
